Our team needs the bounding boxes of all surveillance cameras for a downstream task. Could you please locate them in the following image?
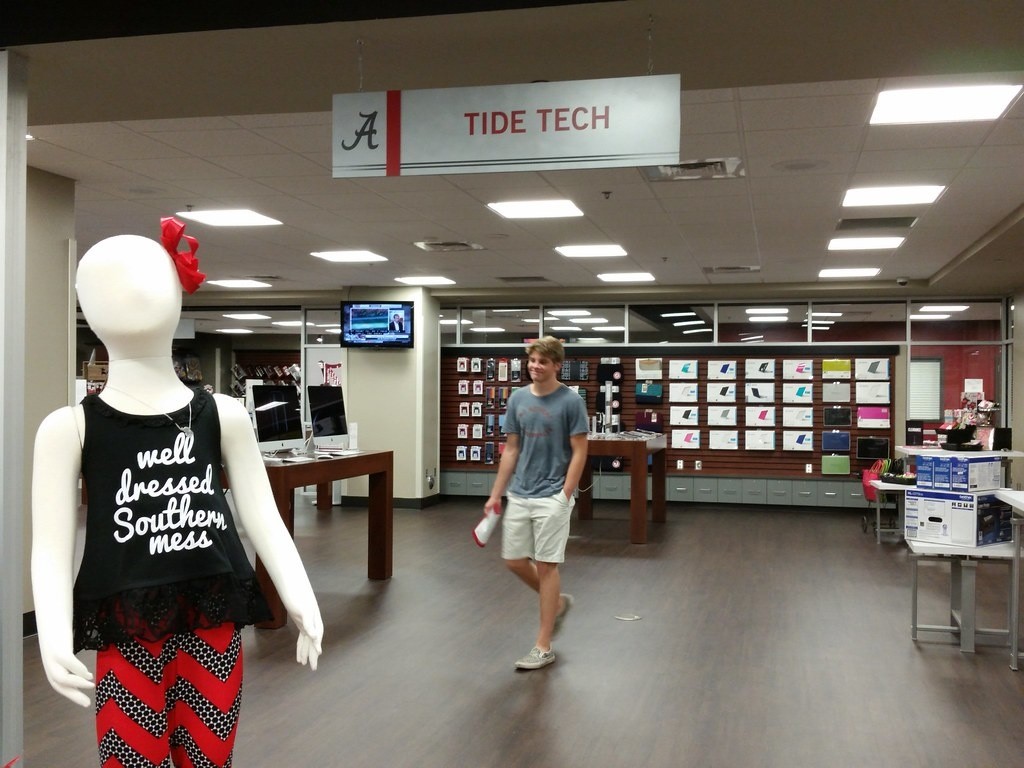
[897,277,909,286]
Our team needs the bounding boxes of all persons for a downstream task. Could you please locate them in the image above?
[31,235,324,768]
[389,314,403,330]
[483,335,591,669]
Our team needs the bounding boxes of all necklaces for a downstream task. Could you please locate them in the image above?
[105,385,193,439]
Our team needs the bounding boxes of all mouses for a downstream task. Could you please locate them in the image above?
[318,454,333,459]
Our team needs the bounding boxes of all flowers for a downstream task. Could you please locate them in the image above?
[160,217,205,295]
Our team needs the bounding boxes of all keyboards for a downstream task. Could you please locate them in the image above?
[282,457,315,463]
[330,451,364,456]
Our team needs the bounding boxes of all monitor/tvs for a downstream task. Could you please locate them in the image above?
[340,301,414,349]
[306,386,349,458]
[252,385,306,458]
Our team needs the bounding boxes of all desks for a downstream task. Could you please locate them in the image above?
[576,433,668,544]
[870,481,917,544]
[218,445,394,630]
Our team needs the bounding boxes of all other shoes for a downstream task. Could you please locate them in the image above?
[548,593,574,641]
[513,647,556,670]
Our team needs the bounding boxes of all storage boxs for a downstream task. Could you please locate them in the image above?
[916,456,1002,493]
[904,490,1013,547]
